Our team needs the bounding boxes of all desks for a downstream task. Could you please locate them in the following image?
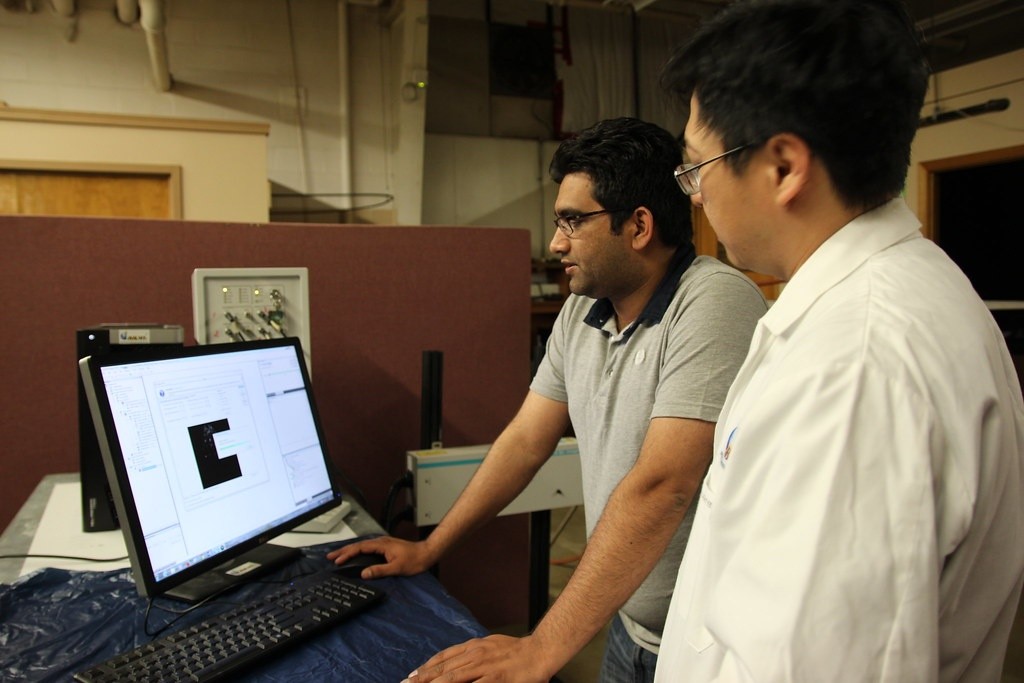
[0,469,493,683]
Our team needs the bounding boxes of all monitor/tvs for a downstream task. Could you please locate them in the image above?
[79,337,343,606]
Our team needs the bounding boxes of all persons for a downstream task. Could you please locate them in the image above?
[650,0,1024,683]
[327,116,770,683]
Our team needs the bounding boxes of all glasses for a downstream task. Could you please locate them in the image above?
[553,210,608,236]
[673,139,758,195]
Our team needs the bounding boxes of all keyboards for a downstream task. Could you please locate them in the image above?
[74,571,383,683]
